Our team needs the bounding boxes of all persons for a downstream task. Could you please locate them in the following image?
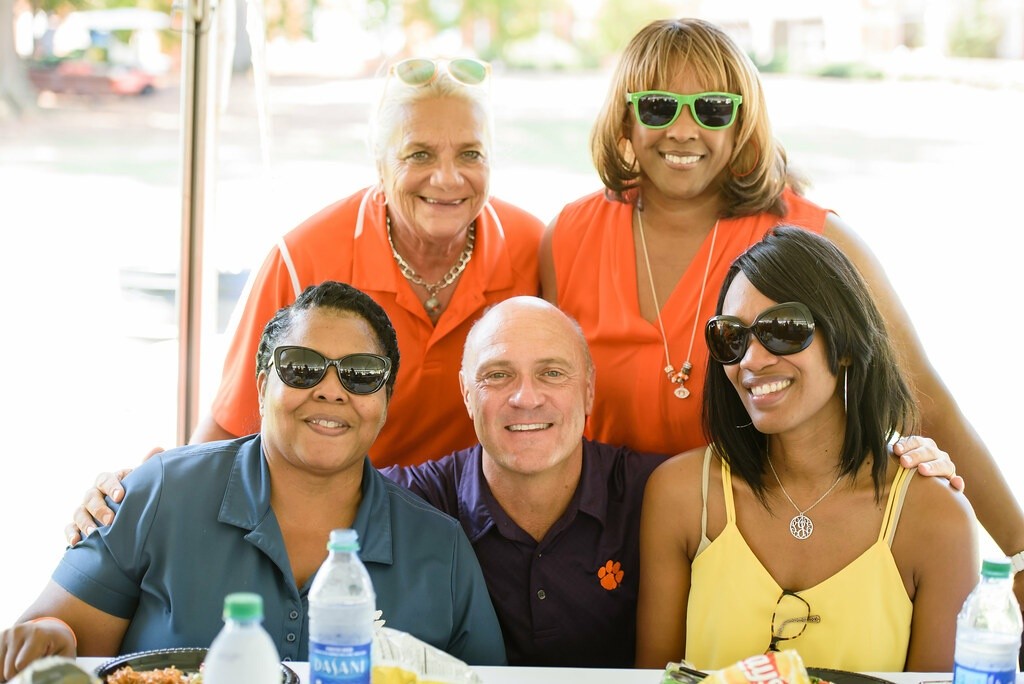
[633,221,980,668]
[67,293,965,672]
[535,15,1024,614]
[2,281,508,681]
[183,58,548,472]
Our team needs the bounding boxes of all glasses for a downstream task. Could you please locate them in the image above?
[389,56,493,97]
[764,590,821,655]
[705,301,820,365]
[627,89,742,128]
[267,345,391,395]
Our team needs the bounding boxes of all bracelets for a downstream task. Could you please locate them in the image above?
[1009,549,1024,575]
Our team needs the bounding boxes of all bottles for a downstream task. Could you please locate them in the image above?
[951,554,1023,683]
[305,527,377,683]
[199,590,285,684]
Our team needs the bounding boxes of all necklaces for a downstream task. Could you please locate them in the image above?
[386,216,476,314]
[637,190,727,399]
[763,446,857,540]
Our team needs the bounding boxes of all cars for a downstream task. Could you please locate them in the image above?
[28,9,181,98]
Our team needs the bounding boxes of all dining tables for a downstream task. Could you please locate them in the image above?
[6,656,1024,684]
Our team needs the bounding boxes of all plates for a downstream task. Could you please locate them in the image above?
[805,665,896,684]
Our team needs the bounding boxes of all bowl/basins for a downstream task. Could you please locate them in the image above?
[90,645,302,684]
